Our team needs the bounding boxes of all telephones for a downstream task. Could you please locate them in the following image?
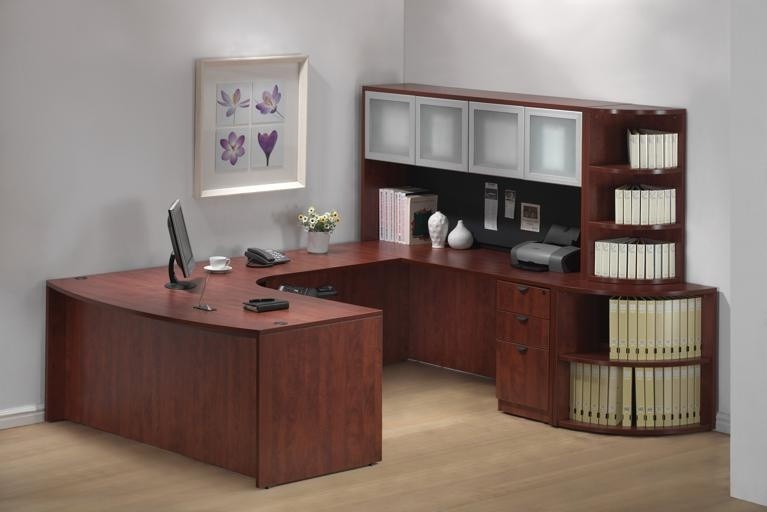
[245,247,291,268]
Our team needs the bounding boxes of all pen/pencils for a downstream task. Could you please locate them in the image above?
[249,298,275,303]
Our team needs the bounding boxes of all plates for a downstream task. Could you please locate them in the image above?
[203,265,233,274]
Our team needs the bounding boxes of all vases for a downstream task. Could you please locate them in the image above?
[306,230,329,253]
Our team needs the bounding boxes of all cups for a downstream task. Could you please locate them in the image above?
[208,255,231,269]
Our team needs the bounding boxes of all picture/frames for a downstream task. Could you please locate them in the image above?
[195,54,308,199]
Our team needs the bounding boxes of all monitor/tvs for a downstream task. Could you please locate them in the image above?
[164,199,198,291]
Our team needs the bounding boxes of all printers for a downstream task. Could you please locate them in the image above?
[510,223,581,273]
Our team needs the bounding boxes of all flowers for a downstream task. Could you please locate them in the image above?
[217,85,286,167]
[297,206,341,233]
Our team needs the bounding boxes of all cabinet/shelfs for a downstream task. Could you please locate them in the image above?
[493,251,556,427]
[401,241,494,381]
[559,282,716,435]
[361,80,687,283]
[360,241,409,365]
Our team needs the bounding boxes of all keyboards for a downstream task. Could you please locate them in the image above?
[278,285,312,296]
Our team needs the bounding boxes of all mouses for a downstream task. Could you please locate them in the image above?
[316,284,334,292]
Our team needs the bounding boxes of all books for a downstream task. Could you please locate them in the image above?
[377,187,439,246]
